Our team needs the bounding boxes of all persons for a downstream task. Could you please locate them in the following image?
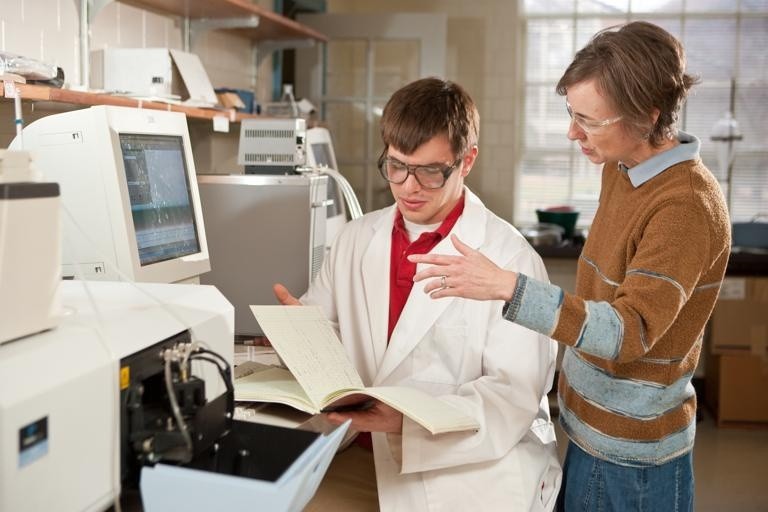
[272,75,566,511]
[407,19,732,511]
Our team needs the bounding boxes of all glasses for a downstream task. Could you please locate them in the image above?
[566,97,625,132]
[377,145,461,189]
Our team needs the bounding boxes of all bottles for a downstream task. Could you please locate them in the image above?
[280,83,298,120]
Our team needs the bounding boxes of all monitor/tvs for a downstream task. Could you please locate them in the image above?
[5,103,213,284]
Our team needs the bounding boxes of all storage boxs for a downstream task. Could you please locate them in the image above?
[704,276,768,429]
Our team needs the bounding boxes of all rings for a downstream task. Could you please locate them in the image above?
[440,276,447,288]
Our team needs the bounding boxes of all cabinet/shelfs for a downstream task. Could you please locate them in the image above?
[6,0,328,129]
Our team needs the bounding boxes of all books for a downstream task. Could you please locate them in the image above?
[231,304,481,436]
